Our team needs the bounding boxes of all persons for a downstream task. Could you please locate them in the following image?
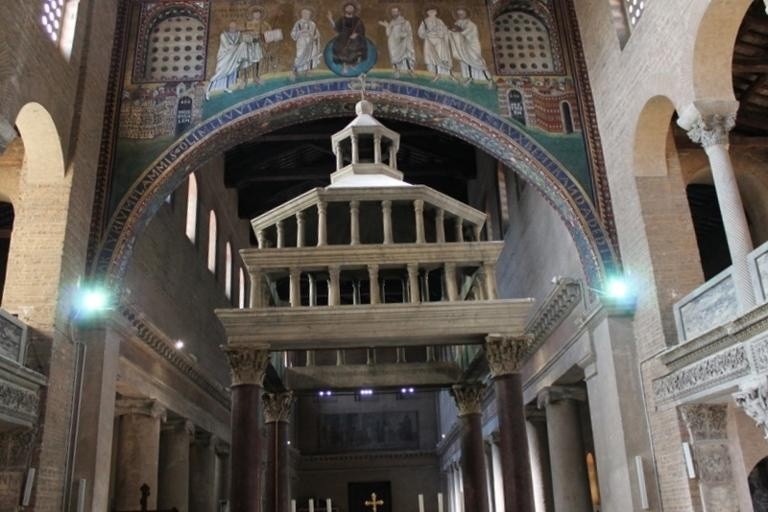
[205,21,249,102]
[447,6,494,90]
[289,7,321,82]
[238,5,273,90]
[325,3,368,76]
[417,5,462,86]
[377,6,421,79]
